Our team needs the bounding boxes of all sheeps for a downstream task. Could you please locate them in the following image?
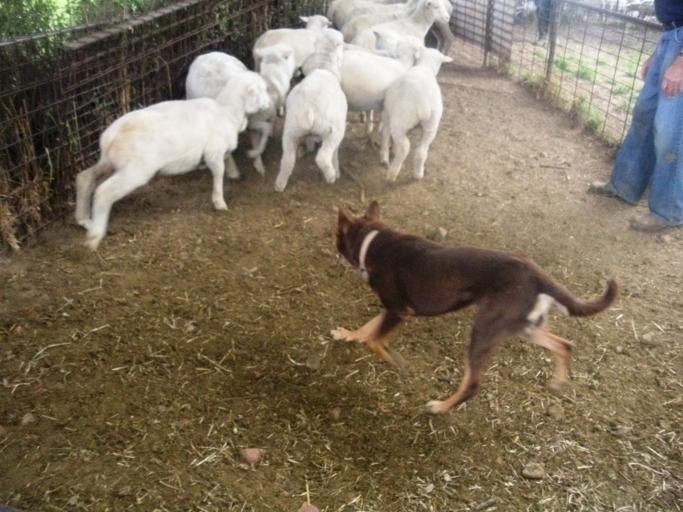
[74,71,272,253]
[253,0,456,192]
[185,43,295,177]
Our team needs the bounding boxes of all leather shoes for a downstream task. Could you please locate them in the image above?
[630,212,667,232]
[590,180,618,198]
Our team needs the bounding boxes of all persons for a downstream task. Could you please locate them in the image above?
[589,0,683,230]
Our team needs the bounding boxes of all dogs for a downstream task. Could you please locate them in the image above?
[330,197,617,416]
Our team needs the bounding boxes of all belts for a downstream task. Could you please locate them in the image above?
[663,18,683,31]
